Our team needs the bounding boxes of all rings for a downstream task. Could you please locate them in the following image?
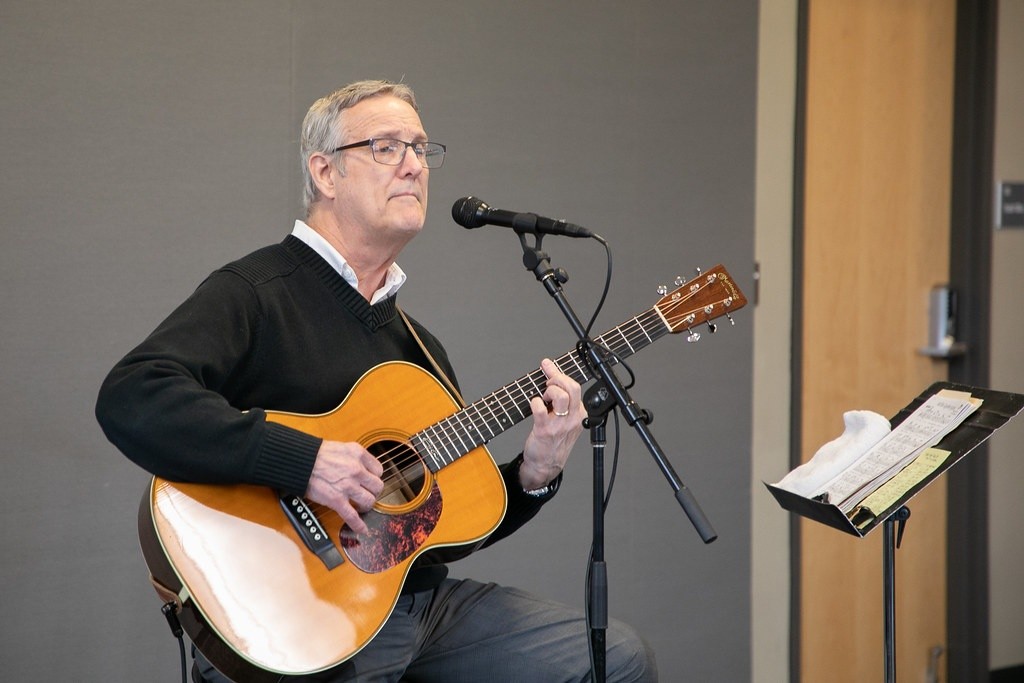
[553,409,569,417]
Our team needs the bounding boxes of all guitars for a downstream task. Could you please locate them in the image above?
[136,261,750,683]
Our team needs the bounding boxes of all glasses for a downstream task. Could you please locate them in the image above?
[327,137,447,169]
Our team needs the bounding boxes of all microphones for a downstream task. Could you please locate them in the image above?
[452,196,592,237]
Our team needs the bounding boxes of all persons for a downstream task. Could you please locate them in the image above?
[93,78,655,683]
